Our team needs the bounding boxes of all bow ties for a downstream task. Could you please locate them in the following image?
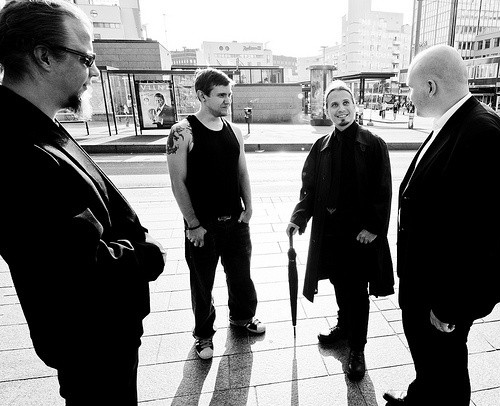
[157,108,161,112]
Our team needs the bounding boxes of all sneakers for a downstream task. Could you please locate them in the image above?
[194,334,214,359]
[229,316,266,333]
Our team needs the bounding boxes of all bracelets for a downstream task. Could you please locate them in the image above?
[187,224,201,230]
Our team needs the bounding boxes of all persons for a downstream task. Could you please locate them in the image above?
[285,80,395,384]
[356,112,374,126]
[0,0,167,406]
[379,99,416,129]
[166,68,266,360]
[148,93,175,127]
[382,42,500,406]
[118,94,140,127]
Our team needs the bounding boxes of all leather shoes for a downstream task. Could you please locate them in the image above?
[347,348,365,381]
[317,326,349,346]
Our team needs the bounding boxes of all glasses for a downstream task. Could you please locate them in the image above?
[59,45,96,67]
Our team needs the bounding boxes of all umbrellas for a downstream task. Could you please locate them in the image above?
[287,227,299,338]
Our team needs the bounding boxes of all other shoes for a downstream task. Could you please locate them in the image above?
[382,391,413,406]
